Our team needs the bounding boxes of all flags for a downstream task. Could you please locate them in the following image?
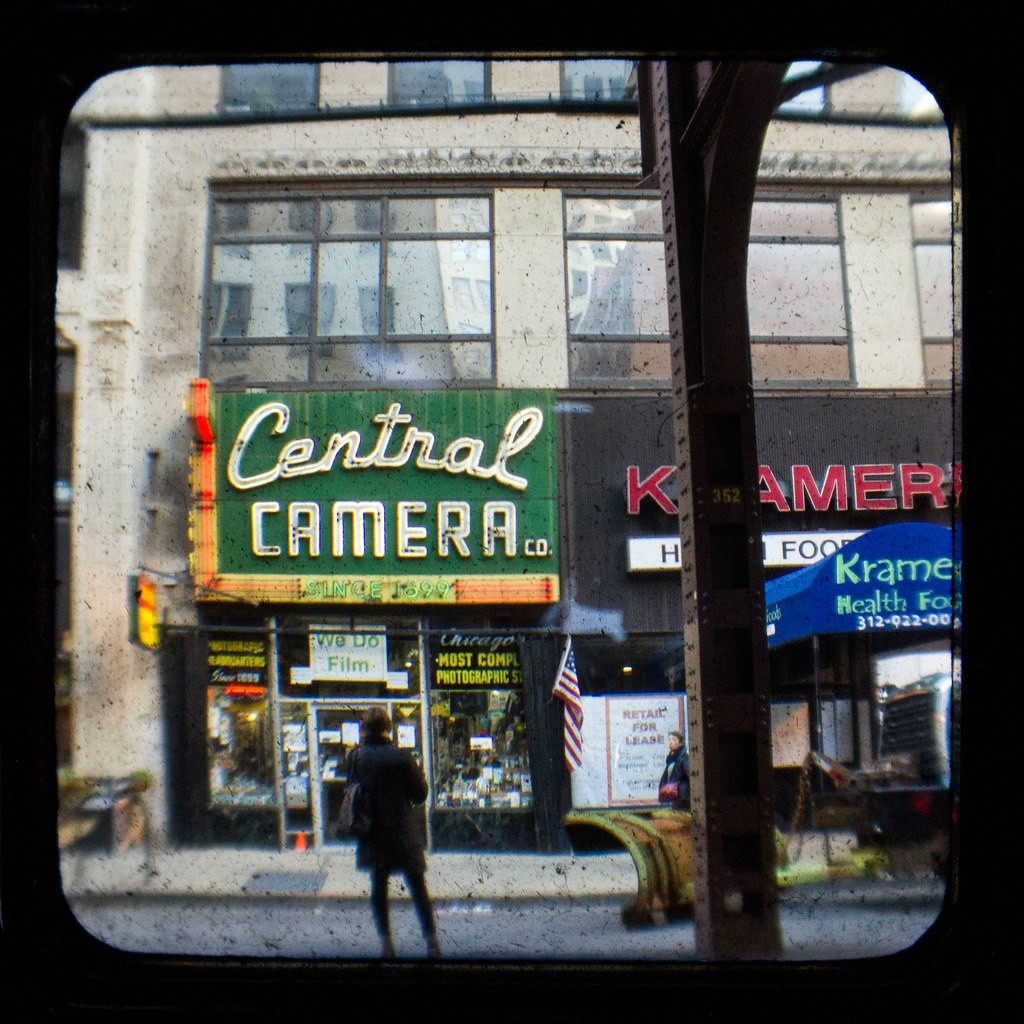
[555,635,583,771]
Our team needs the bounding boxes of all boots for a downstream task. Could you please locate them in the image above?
[379,935,395,959]
[423,934,443,959]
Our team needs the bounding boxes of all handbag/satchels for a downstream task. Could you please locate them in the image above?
[334,746,370,840]
[658,783,678,802]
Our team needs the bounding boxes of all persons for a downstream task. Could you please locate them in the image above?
[347,707,441,959]
[659,731,693,812]
